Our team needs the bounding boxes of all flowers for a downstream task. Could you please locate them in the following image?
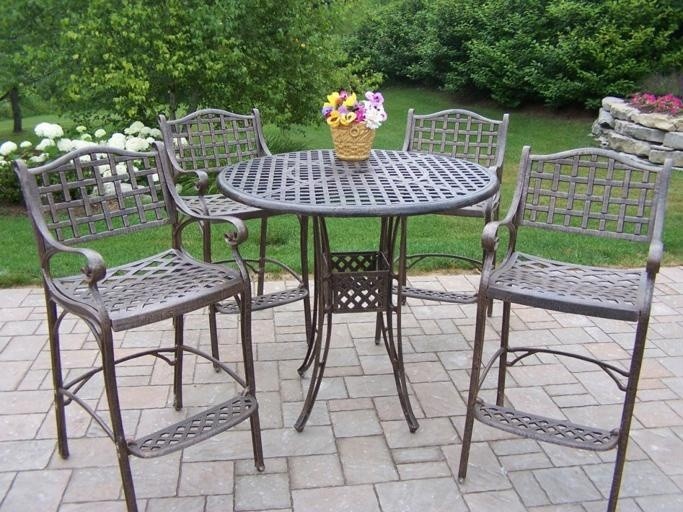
[323,87,387,130]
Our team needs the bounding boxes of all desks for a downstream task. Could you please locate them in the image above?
[217,146,500,435]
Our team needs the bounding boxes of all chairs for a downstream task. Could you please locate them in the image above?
[458,145,673,512]
[155,108,316,376]
[373,106,513,356]
[11,138,267,512]
